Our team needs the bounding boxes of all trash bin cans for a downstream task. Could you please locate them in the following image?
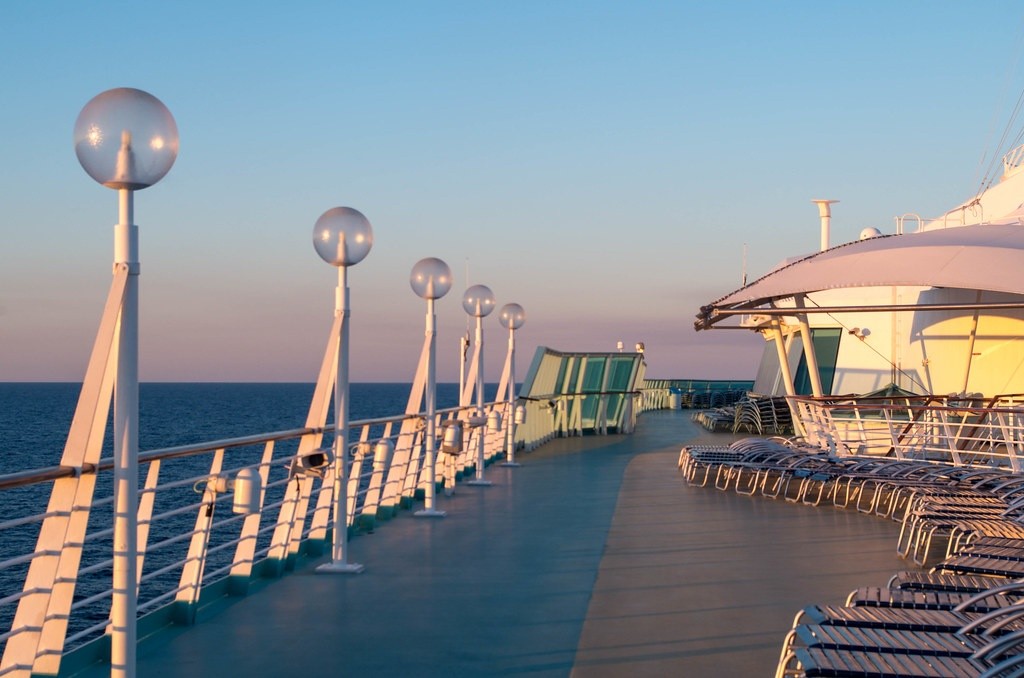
[668,387,682,410]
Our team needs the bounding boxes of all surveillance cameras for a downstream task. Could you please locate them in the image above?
[849,328,860,335]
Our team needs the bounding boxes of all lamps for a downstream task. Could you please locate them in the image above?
[209,469,262,515]
[488,411,502,433]
[372,439,394,468]
[558,400,565,411]
[514,405,526,424]
[444,424,460,455]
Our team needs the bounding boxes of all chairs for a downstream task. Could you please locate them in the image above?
[681,387,792,435]
[676,436,1024,678]
